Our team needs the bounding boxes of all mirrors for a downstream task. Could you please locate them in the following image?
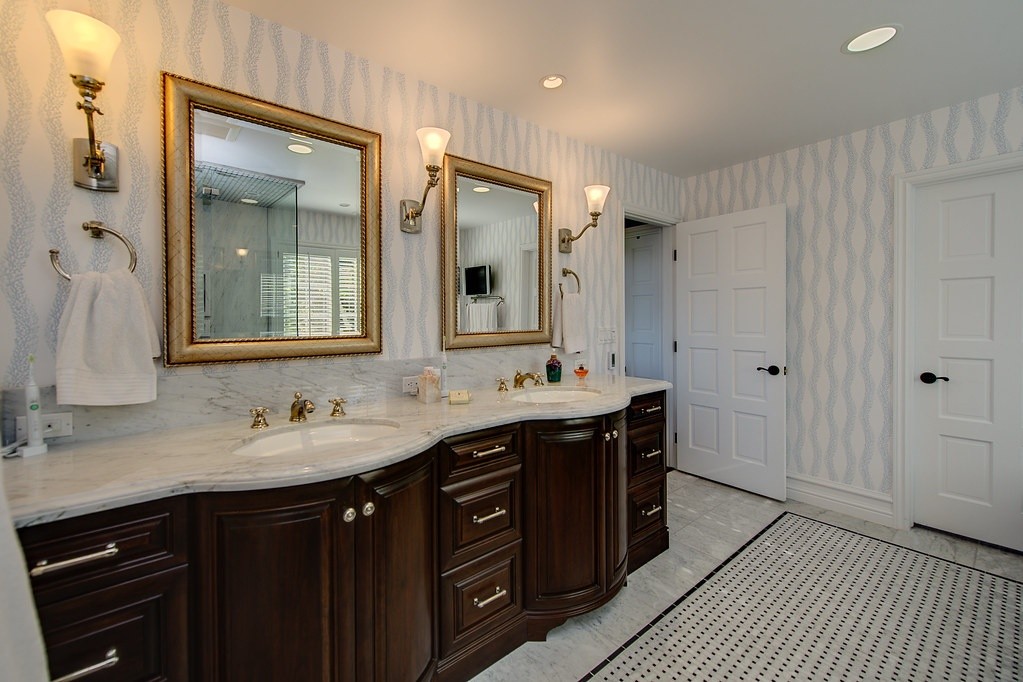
[457,175,541,333]
[193,109,362,335]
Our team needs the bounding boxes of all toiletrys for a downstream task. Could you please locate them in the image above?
[572,358,591,380]
[546,346,563,384]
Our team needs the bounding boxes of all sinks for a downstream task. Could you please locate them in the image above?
[505,385,604,404]
[227,417,402,459]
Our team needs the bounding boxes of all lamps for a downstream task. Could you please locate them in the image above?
[46,8,122,180]
[407,127,451,218]
[565,185,610,242]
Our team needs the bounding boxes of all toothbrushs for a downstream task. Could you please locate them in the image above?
[440,335,450,391]
[24,353,44,448]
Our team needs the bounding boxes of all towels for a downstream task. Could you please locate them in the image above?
[549,292,590,357]
[464,302,500,334]
[56,265,162,408]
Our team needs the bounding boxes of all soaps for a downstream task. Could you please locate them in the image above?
[447,388,471,406]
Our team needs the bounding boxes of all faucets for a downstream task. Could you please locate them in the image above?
[288,391,316,423]
[513,367,544,391]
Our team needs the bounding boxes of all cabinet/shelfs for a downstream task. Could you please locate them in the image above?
[188,442,440,682]
[438,422,524,682]
[524,409,628,641]
[628,390,669,575]
[18,495,188,682]
